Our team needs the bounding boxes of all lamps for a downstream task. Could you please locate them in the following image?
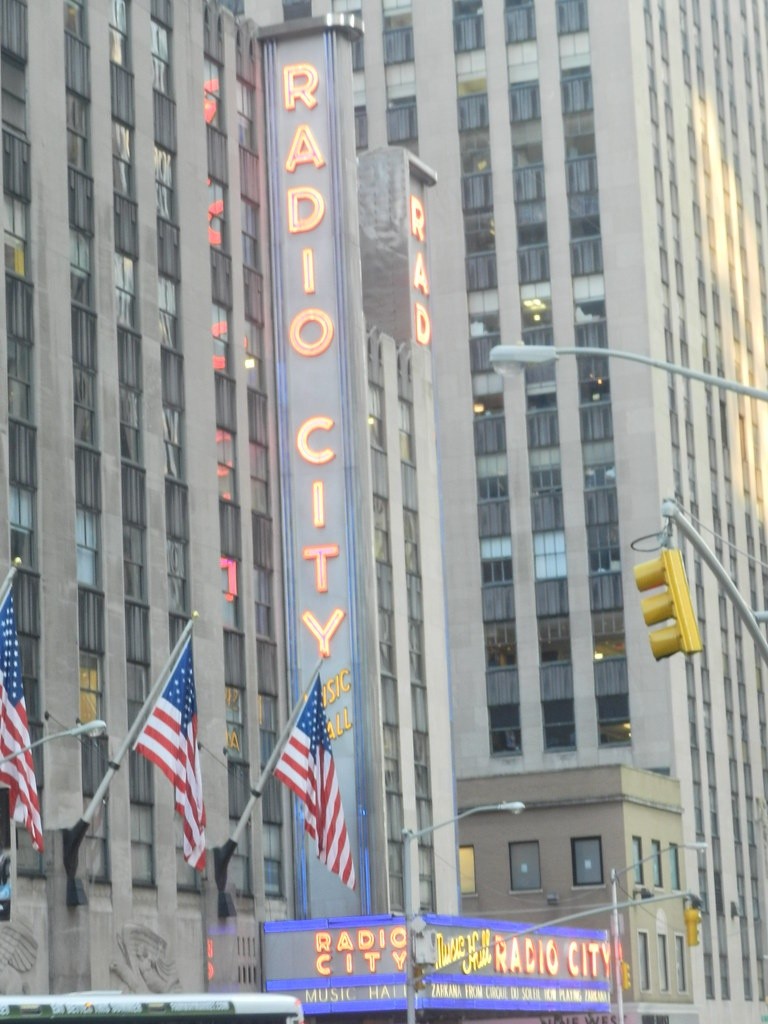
[547,893,560,905]
[633,889,654,903]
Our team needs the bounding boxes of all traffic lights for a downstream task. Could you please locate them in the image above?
[684,907,703,946]
[633,547,703,662]
[620,962,632,991]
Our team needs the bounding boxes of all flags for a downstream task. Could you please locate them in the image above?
[273,674,356,891]
[133,637,208,871]
[0,584,45,853]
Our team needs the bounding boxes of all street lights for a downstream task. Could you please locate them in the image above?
[401,800,528,1024]
[609,841,709,1024]
